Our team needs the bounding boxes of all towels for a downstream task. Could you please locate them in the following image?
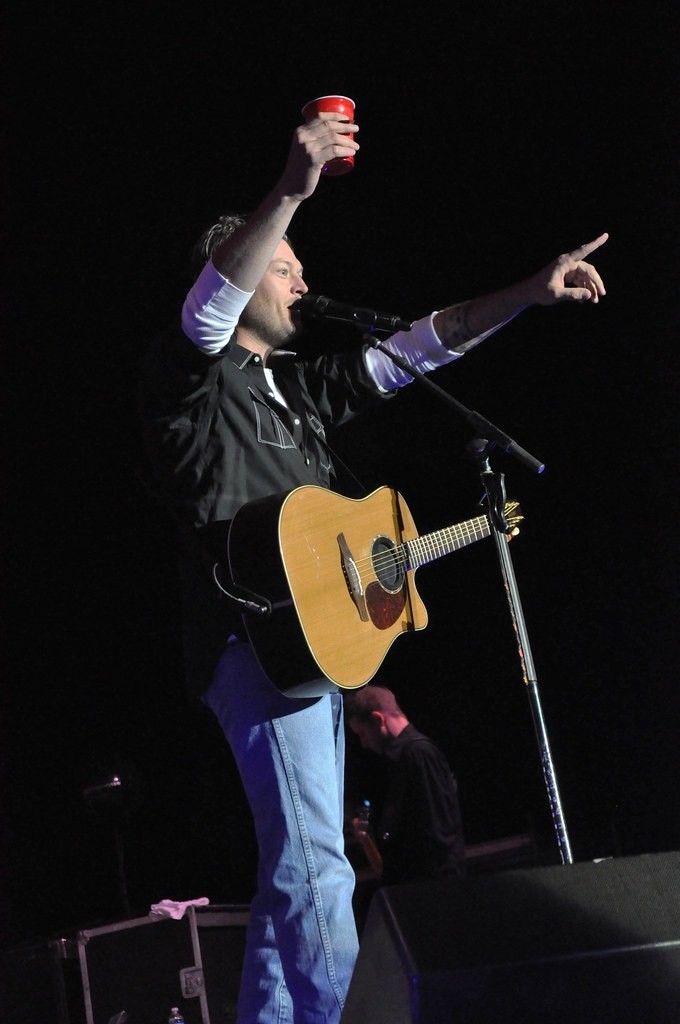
[149,897,209,922]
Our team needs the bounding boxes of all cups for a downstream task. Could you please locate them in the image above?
[300,95,356,176]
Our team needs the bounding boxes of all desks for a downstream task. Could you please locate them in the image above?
[76,904,250,1024]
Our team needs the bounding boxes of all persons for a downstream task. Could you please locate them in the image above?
[136,111,611,1021]
[346,686,467,887]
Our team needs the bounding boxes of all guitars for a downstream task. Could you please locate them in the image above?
[225,483,527,692]
